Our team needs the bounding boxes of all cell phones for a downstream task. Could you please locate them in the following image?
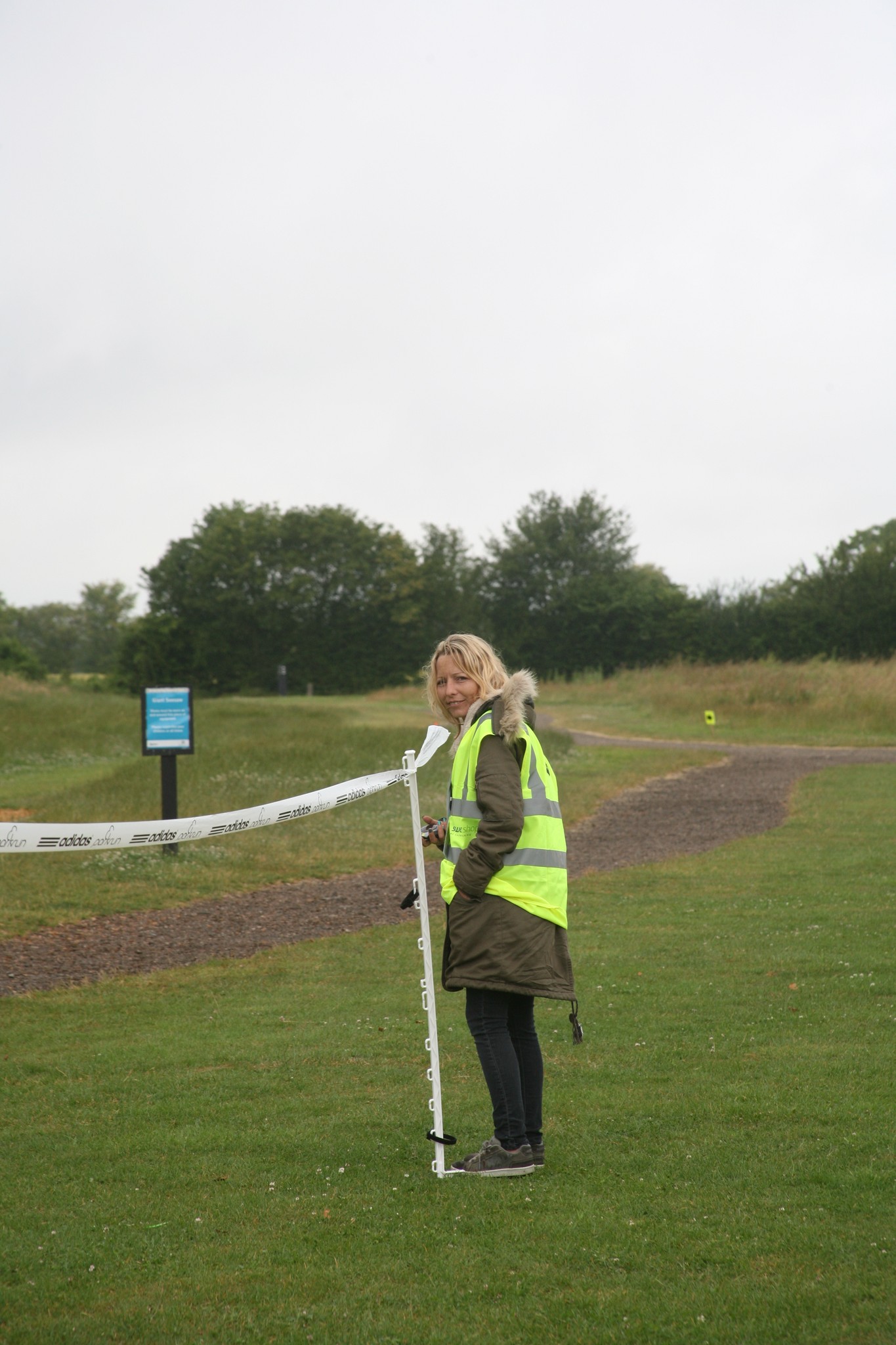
[420,818,447,838]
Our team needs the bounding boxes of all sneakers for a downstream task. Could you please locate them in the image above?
[462,1139,545,1167]
[449,1138,535,1178]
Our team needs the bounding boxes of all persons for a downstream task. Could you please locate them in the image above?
[419,633,583,1177]
